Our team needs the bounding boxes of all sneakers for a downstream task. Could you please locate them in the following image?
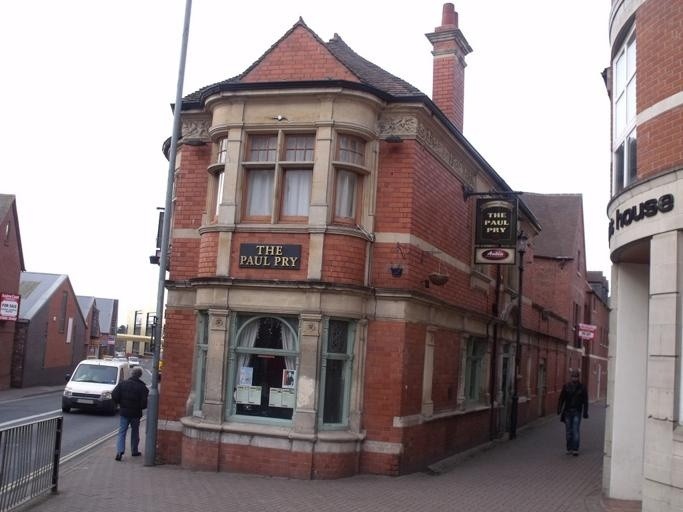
[567,450,578,457]
[117,451,139,463]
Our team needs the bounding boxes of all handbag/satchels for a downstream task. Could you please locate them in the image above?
[559,410,568,424]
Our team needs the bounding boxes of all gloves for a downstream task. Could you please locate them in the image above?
[558,410,562,415]
[583,414,588,419]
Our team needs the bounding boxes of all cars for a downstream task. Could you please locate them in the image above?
[62,351,140,416]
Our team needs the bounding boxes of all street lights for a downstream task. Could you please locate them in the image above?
[510,230,529,439]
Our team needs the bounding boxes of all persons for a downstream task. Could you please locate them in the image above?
[111,366,149,461]
[557,371,589,456]
